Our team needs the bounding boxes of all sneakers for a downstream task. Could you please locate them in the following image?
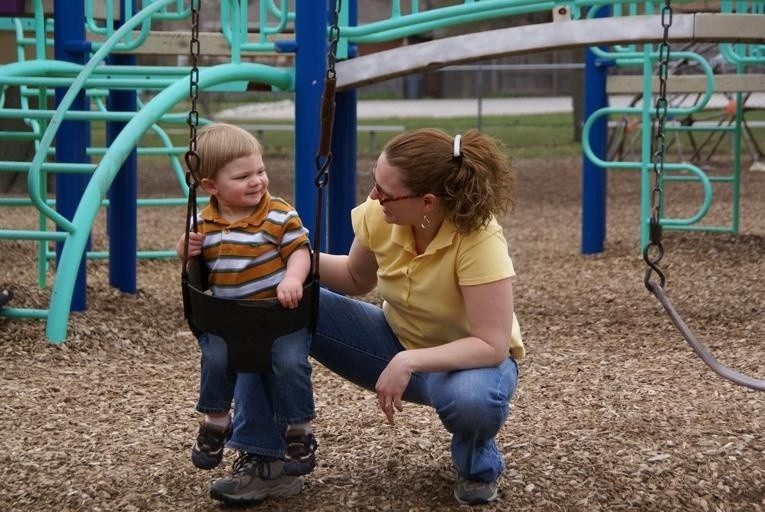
[454,470,498,504]
[192,413,317,501]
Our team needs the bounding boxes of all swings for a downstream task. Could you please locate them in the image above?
[644,0,765,391]
[181,0,341,375]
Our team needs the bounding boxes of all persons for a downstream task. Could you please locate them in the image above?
[175,123,319,477]
[210,126,527,507]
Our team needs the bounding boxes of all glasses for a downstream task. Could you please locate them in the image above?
[373,164,441,205]
